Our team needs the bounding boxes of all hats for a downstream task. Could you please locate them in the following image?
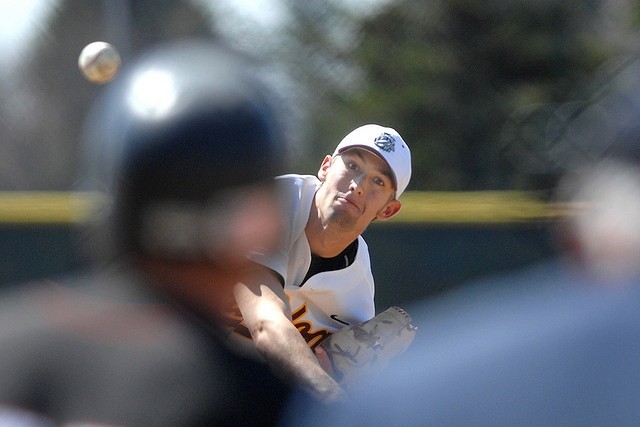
[332,123,414,202]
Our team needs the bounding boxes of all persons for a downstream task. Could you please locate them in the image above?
[0,44,289,427]
[221,123,413,424]
[288,64,640,426]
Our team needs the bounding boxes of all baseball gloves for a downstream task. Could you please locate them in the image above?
[322,306,418,387]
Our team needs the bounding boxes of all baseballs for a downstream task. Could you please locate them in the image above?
[77,41,121,86]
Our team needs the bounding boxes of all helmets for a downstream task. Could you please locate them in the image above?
[107,35,287,265]
